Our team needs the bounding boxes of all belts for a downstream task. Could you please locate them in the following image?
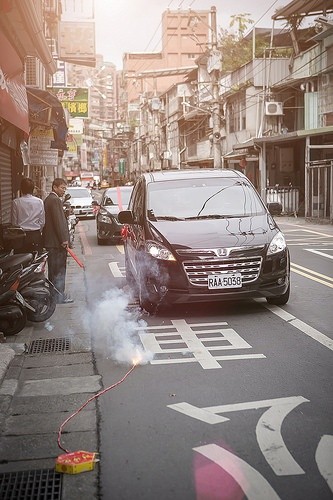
[25,230,40,233]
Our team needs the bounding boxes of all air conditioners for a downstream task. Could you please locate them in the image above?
[265,101,283,115]
[23,54,46,90]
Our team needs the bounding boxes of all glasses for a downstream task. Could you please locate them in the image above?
[59,186,66,190]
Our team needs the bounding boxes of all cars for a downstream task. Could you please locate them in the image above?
[61,186,95,219]
[92,186,135,244]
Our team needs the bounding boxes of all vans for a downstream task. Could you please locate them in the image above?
[118,167,291,314]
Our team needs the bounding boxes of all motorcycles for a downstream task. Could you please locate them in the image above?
[60,193,80,249]
[0,223,56,337]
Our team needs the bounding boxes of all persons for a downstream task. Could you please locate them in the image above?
[43,177,74,303]
[11,177,45,263]
[86,181,112,190]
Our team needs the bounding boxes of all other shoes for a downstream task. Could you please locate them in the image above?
[57,298,74,303]
